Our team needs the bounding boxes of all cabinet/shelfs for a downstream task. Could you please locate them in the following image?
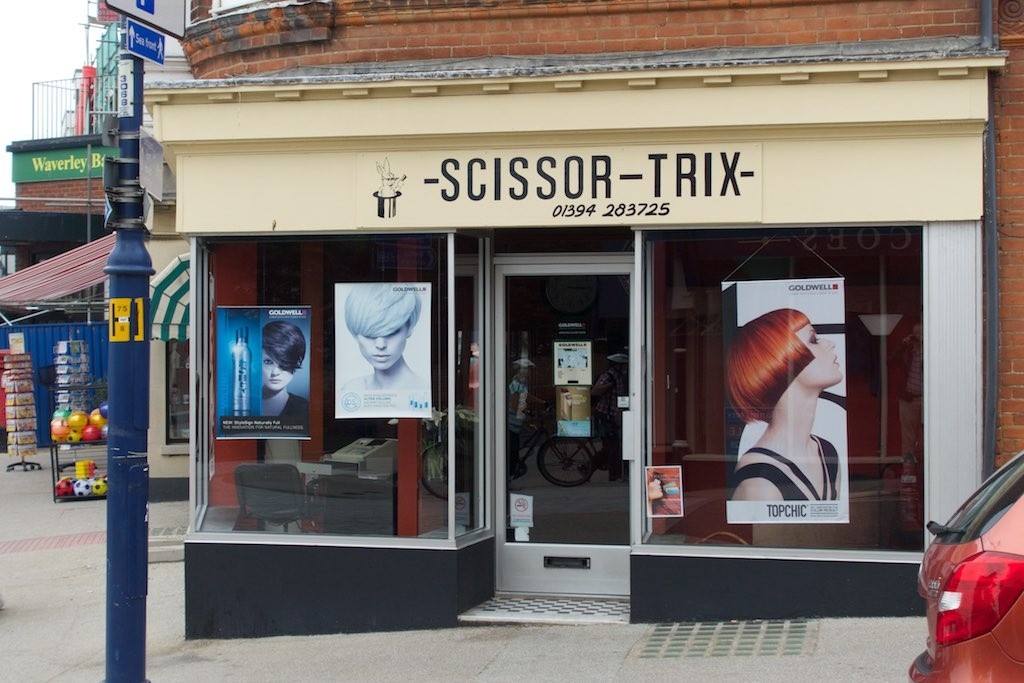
[47,381,112,503]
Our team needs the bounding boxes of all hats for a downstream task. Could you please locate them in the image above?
[511,359,534,369]
[607,352,629,363]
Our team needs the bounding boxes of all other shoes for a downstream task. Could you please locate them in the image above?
[509,476,520,490]
[577,462,618,483]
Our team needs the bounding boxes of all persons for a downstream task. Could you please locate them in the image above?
[886,318,923,476]
[261,320,310,416]
[165,338,189,437]
[724,308,844,502]
[338,283,429,397]
[578,352,629,486]
[647,469,667,515]
[508,359,553,483]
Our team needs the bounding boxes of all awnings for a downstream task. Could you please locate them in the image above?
[0,231,119,301]
[148,253,190,341]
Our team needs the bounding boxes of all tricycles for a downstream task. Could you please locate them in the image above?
[419,383,593,499]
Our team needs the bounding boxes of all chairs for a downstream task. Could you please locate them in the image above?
[233,462,307,534]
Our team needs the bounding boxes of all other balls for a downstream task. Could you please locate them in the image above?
[49,398,110,497]
